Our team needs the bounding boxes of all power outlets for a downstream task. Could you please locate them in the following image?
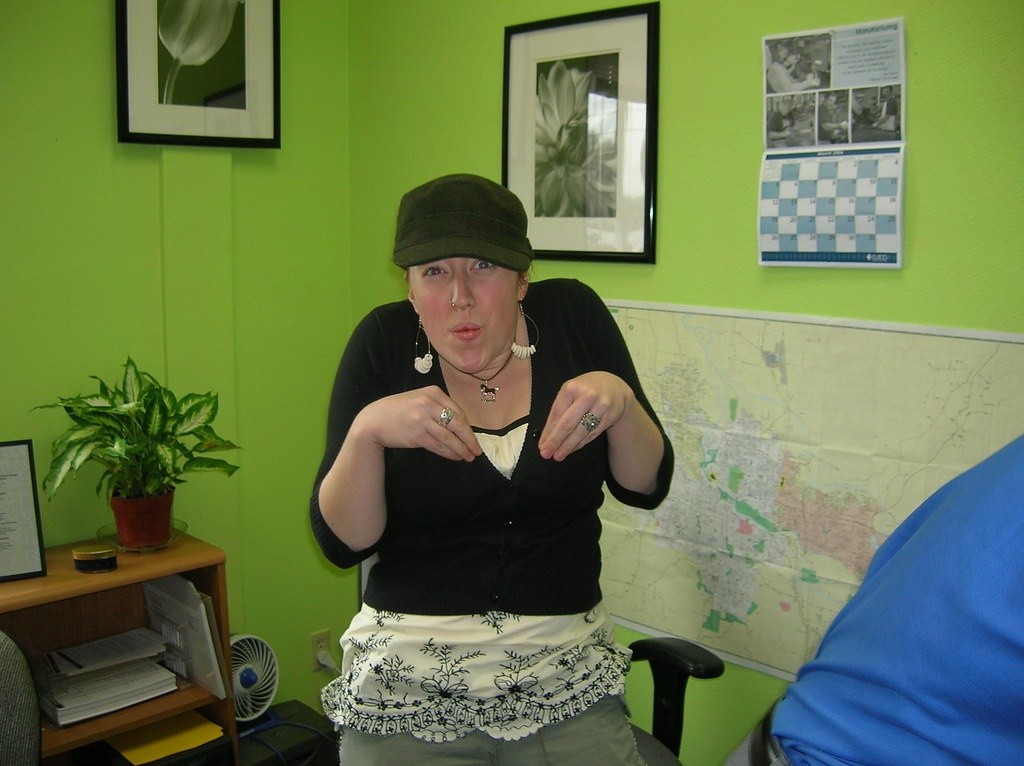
[310,628,332,672]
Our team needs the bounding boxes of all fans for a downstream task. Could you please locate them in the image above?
[230,633,279,721]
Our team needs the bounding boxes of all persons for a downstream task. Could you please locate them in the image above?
[720,434,1024,766]
[768,100,815,148]
[310,173,674,766]
[766,44,821,93]
[866,86,898,130]
[818,92,849,145]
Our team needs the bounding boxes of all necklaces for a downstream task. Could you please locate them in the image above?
[469,333,516,404]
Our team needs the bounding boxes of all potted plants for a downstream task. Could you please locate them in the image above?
[24,354,244,553]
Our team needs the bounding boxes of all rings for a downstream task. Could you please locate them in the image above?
[581,412,601,431]
[438,406,455,428]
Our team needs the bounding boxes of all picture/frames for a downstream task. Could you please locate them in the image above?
[0,439,47,583]
[502,1,660,264]
[113,0,283,150]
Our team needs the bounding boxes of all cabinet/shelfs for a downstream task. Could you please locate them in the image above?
[0,529,241,766]
[239,700,340,766]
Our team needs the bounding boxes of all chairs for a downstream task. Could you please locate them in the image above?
[628,637,726,766]
[0,630,41,766]
